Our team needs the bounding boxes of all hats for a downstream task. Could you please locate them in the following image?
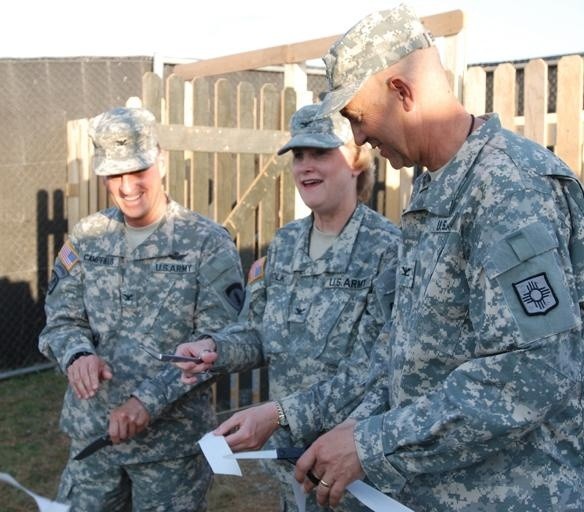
[87,106,158,178]
[277,105,354,156]
[312,2,437,122]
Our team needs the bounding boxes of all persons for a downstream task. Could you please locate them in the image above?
[172,104,403,510]
[36,107,247,510]
[294,3,584,512]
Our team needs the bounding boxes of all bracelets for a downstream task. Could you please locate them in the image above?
[67,351,92,367]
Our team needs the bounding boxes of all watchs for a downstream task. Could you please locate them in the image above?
[277,401,290,432]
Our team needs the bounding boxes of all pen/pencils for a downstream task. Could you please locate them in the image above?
[159,353,203,363]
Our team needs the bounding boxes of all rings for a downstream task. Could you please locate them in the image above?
[321,480,330,487]
